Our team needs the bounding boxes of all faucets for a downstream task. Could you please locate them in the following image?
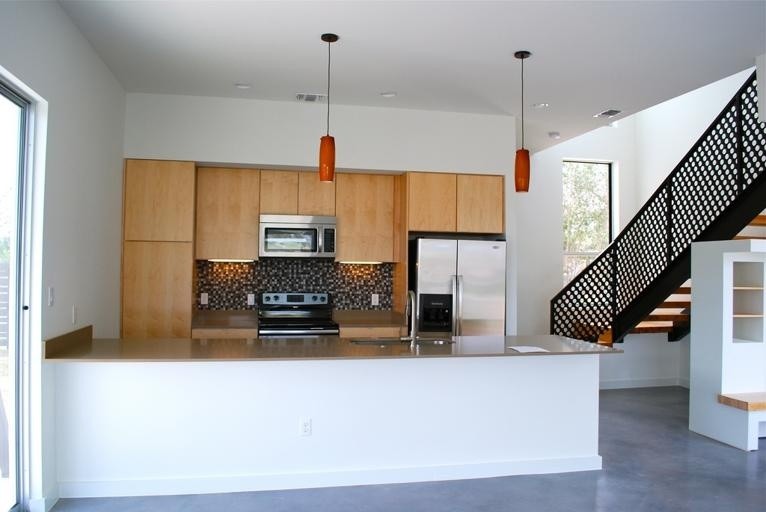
[407,288,418,339]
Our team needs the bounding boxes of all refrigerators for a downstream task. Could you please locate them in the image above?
[408,236,506,339]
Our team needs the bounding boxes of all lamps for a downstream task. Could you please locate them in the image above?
[319,32,337,186]
[513,49,531,193]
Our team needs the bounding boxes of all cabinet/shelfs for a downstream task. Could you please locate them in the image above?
[260,171,338,215]
[335,175,399,261]
[405,171,503,236]
[196,167,260,261]
[123,156,196,242]
[687,237,766,454]
[120,242,194,340]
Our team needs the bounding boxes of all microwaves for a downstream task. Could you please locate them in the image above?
[256,212,337,261]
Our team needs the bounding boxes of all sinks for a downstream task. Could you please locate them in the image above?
[349,338,453,347]
